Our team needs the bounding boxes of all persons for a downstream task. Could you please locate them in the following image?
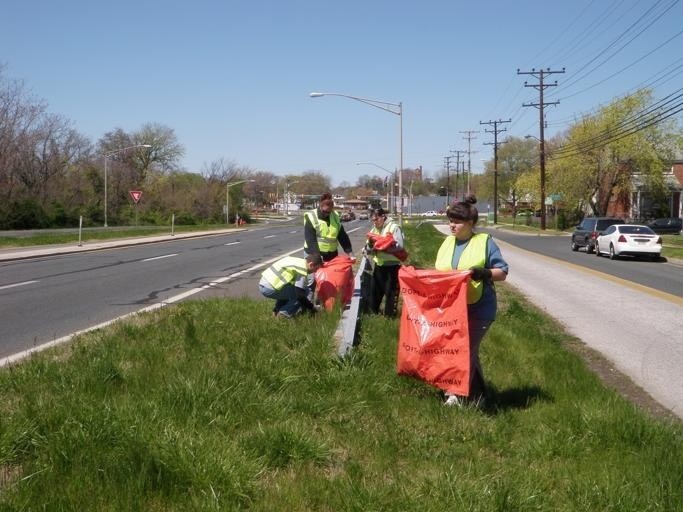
[258,251,322,320]
[303,192,356,303]
[365,206,404,315]
[406,194,508,405]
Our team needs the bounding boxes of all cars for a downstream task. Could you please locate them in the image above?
[518,208,556,218]
[329,207,370,226]
[595,224,662,259]
[420,210,437,217]
[633,218,682,236]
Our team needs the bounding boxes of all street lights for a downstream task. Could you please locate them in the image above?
[224,179,255,226]
[308,92,406,229]
[440,185,449,207]
[480,159,499,223]
[525,134,547,232]
[269,180,279,215]
[355,159,398,182]
[284,180,300,216]
[99,145,149,226]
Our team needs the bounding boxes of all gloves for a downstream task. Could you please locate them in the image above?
[469,266,493,282]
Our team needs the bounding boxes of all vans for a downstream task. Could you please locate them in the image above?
[569,215,624,256]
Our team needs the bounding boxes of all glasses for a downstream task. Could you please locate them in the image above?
[321,209,333,215]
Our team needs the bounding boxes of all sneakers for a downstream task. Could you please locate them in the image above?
[276,309,294,320]
[441,392,464,409]
